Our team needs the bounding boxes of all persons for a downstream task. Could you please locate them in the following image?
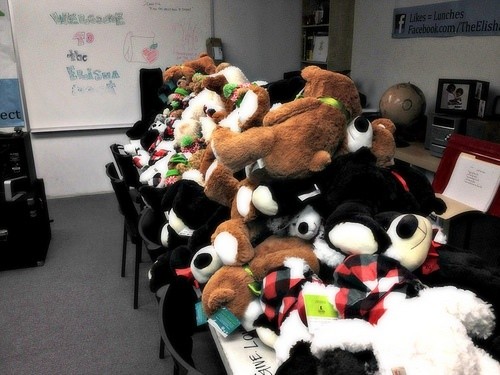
[445,84,464,112]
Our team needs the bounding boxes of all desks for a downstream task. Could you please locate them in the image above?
[393,139,442,173]
[195,282,284,375]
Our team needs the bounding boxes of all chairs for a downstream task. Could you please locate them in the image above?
[431,133,500,219]
[104,144,228,375]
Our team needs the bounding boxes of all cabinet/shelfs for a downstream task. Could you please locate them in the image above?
[301,0,356,72]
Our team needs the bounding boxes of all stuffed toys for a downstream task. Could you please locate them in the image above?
[124,50,500,375]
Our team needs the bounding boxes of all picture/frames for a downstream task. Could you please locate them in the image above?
[435,79,490,120]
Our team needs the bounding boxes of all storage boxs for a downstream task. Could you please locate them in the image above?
[0,132,52,272]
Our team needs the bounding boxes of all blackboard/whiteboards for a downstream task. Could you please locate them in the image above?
[8,1,216,133]
